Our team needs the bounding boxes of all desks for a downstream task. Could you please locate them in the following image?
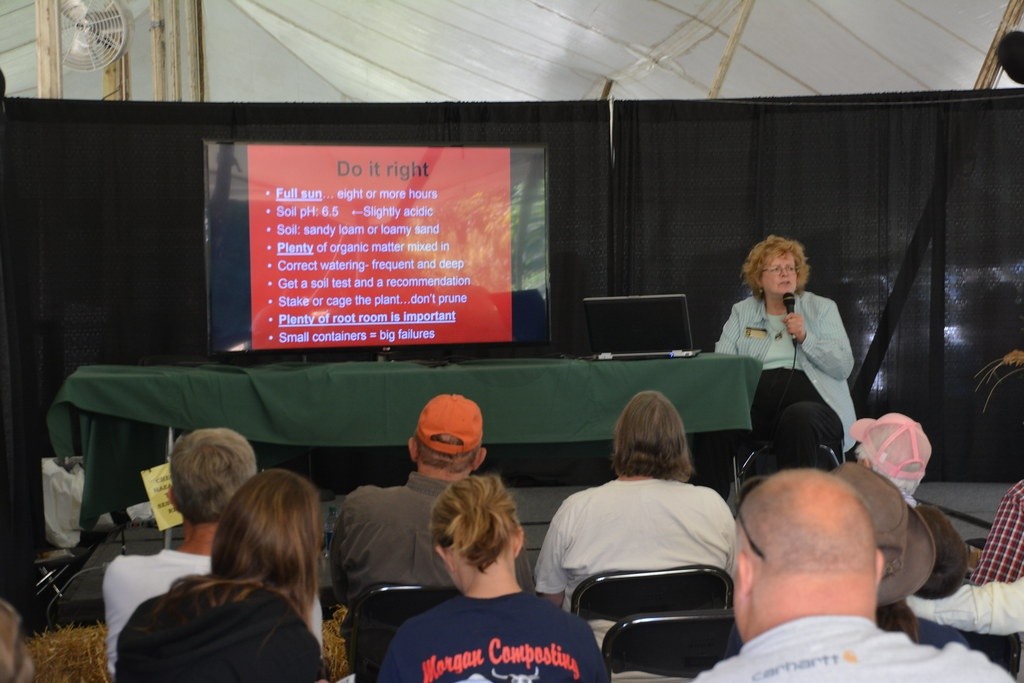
[46,353,764,552]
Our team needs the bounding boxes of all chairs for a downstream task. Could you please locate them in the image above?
[600,609,743,683]
[569,564,735,623]
[346,581,458,683]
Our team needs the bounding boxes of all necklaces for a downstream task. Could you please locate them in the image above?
[763,313,789,342]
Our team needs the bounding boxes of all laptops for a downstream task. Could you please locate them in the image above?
[583,294,702,360]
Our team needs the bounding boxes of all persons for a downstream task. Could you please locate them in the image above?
[102,426,324,683]
[329,393,537,611]
[377,474,610,683]
[691,402,1024,683]
[114,468,330,683]
[715,235,861,465]
[0,597,35,683]
[533,390,738,683]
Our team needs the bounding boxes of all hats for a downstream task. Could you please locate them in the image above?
[851,413,932,481]
[416,393,483,454]
[830,463,937,608]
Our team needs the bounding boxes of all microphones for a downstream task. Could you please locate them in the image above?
[783,293,797,348]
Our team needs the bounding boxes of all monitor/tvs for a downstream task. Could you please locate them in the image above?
[202,140,554,357]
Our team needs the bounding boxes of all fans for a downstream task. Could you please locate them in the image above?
[57,0,137,73]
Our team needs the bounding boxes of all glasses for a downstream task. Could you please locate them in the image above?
[761,265,801,274]
[734,476,767,560]
